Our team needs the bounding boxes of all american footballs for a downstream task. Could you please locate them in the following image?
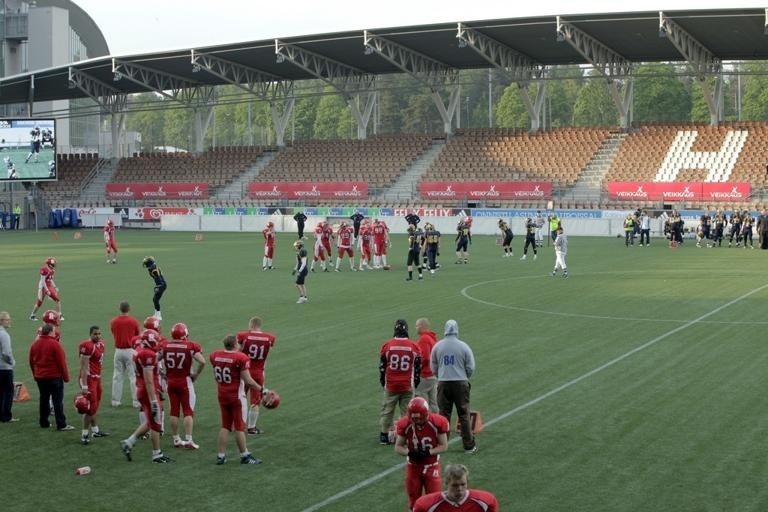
[382,264,391,271]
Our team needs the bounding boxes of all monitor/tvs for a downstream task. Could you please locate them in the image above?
[0,118,59,182]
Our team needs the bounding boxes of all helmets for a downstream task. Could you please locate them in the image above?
[140,330,161,352]
[317,223,322,227]
[171,323,188,340]
[75,393,90,413]
[108,220,113,226]
[407,397,429,419]
[361,219,369,225]
[496,218,503,224]
[394,319,408,334]
[321,221,328,229]
[458,219,466,225]
[445,320,458,335]
[340,221,347,227]
[42,311,61,327]
[407,225,415,233]
[144,317,160,330]
[294,241,304,247]
[267,222,274,228]
[524,218,532,223]
[46,257,56,267]
[425,224,433,229]
[424,223,428,227]
[141,256,155,268]
[416,228,422,232]
[373,219,378,223]
[261,390,280,409]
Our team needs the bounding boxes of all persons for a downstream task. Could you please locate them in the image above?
[728,210,743,248]
[292,241,309,304]
[421,247,430,272]
[695,212,712,249]
[455,222,472,265]
[335,222,357,273]
[34,310,61,344]
[405,224,425,281]
[104,219,118,265]
[322,220,335,267]
[29,324,76,431]
[553,228,568,279]
[48,160,56,177]
[24,127,40,163]
[3,157,16,179]
[710,208,728,248]
[236,317,275,436]
[548,213,561,241]
[413,463,500,512]
[429,319,479,455]
[756,207,768,249]
[29,257,65,321]
[294,211,307,240]
[359,225,373,271]
[209,335,268,466]
[142,256,167,320]
[420,222,439,274]
[533,211,545,248]
[120,329,177,465]
[670,214,684,244]
[741,213,755,249]
[138,316,168,440]
[110,301,143,408]
[0,310,21,422]
[638,210,651,247]
[262,222,276,271]
[41,127,55,149]
[79,326,111,444]
[13,203,21,231]
[405,213,420,238]
[415,317,439,414]
[158,322,206,450]
[632,212,642,239]
[371,219,392,269]
[309,222,328,272]
[519,218,537,261]
[395,396,450,511]
[623,214,635,248]
[350,209,364,241]
[378,320,421,445]
[498,219,513,258]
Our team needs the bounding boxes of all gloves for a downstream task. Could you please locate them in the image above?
[418,451,429,461]
[380,375,385,386]
[414,378,419,388]
[408,448,416,459]
[292,270,296,275]
[296,271,300,278]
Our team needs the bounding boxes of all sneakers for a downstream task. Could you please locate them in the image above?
[646,243,649,247]
[296,297,304,304]
[155,311,161,319]
[360,267,363,270]
[533,254,537,260]
[30,314,37,321]
[380,431,389,444]
[182,439,198,450]
[706,244,710,248]
[350,267,358,272]
[112,399,120,406]
[640,244,643,247]
[153,453,175,463]
[713,243,715,246]
[744,247,746,248]
[719,244,721,246]
[561,271,568,277]
[215,454,226,465]
[736,243,739,246]
[322,268,329,272]
[329,262,333,266]
[696,244,700,247]
[510,253,512,256]
[248,426,264,436]
[269,267,274,269]
[503,253,508,256]
[7,418,20,421]
[374,265,378,268]
[729,243,731,247]
[91,430,110,438]
[121,440,134,462]
[418,276,423,279]
[142,433,149,438]
[464,259,469,263]
[429,270,434,273]
[132,401,141,406]
[335,268,341,272]
[520,254,526,260]
[321,264,322,267]
[422,264,426,269]
[455,259,462,263]
[113,259,117,263]
[750,246,753,248]
[240,452,262,466]
[625,245,628,246]
[45,423,52,427]
[262,267,268,271]
[630,243,633,246]
[553,271,555,275]
[310,267,317,272]
[81,436,90,443]
[106,260,111,263]
[378,265,382,268]
[366,265,372,269]
[175,440,184,446]
[60,424,75,431]
[465,445,478,455]
[406,277,411,280]
[305,298,307,302]
